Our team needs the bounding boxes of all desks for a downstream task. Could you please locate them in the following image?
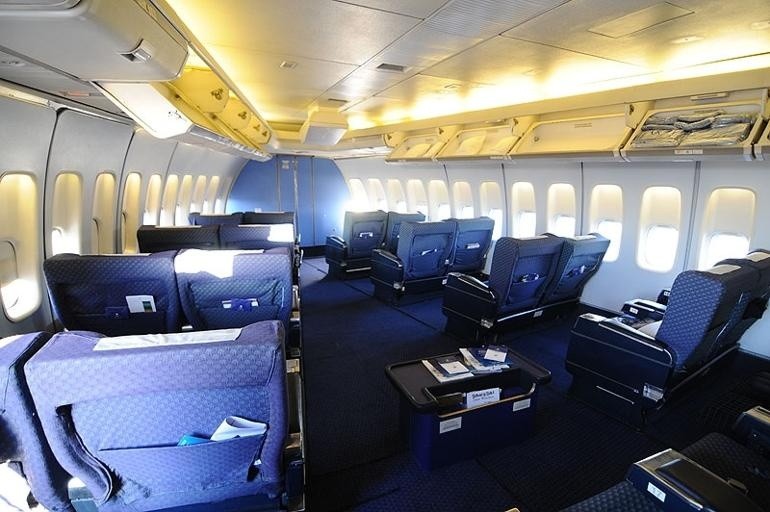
[383,340,553,475]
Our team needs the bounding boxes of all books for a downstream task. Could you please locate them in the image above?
[422,342,518,383]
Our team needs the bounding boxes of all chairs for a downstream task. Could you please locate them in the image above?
[564,248,770,431]
[440,232,611,347]
[501,400,770,511]
[323,209,426,279]
[1,318,308,511]
[136,212,302,284]
[369,215,496,307]
[43,247,303,359]
